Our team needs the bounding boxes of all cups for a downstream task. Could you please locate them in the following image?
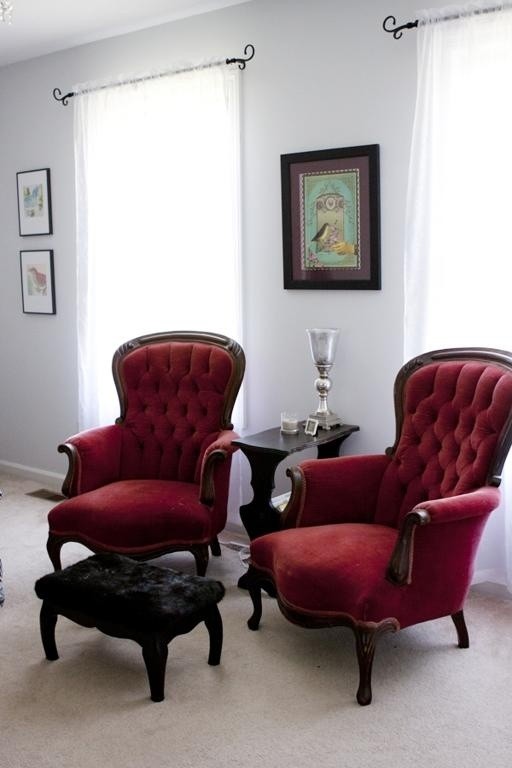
[280,410,299,435]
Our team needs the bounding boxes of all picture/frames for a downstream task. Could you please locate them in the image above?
[16,168,52,237]
[20,250,55,314]
[279,144,382,290]
